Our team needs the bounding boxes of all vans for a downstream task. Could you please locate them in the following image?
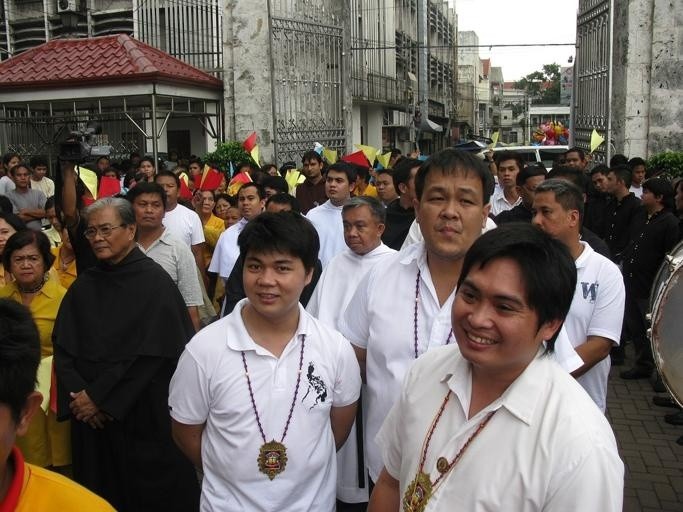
[479,146,571,171]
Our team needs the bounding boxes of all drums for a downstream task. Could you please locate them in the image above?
[648,242,683,410]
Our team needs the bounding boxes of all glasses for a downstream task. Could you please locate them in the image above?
[83,222,131,240]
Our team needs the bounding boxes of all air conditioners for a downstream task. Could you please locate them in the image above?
[57,0,81,14]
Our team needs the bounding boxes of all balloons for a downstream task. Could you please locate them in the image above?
[531,119,569,146]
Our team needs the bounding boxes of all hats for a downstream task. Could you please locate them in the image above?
[284,161,296,170]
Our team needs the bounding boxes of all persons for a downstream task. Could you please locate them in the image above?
[0,148,683,511]
